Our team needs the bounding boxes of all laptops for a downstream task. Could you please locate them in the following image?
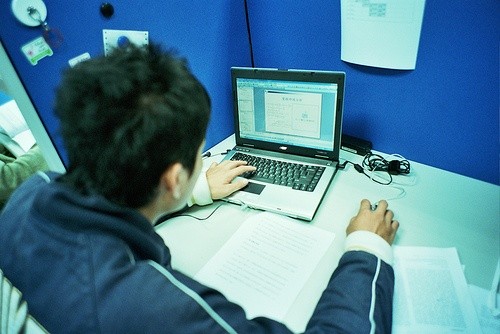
[218,66,346,222]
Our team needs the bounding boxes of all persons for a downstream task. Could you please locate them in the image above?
[0,45,400,334]
[0,142,51,207]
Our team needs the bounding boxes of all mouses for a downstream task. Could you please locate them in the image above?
[370,205,377,211]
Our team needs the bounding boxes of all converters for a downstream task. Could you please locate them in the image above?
[340,133,372,156]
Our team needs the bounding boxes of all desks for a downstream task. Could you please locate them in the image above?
[154,134,500,334]
